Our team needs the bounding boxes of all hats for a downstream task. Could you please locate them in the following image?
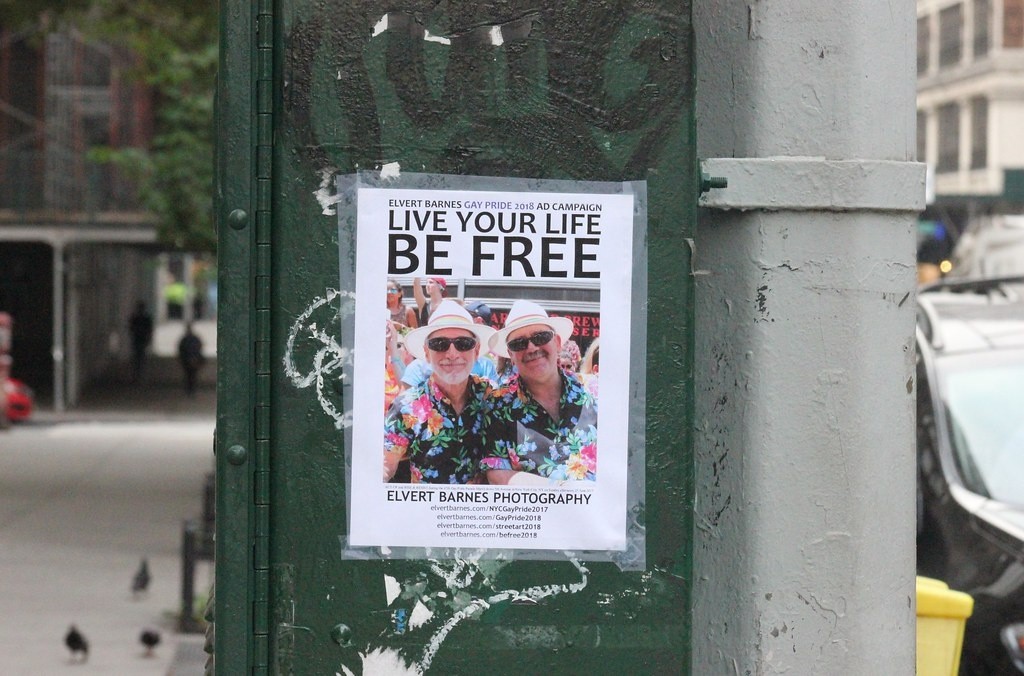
[403,301,498,358]
[431,278,448,298]
[465,300,492,323]
[488,301,573,358]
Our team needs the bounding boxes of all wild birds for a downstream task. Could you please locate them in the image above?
[139,629,160,652]
[65,622,88,662]
[130,558,151,601]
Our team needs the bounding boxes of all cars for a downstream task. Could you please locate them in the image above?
[915,292,1024,674]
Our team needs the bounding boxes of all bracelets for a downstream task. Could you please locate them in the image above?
[552,480,566,486]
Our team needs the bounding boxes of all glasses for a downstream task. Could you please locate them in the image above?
[427,335,479,352]
[561,364,573,370]
[387,289,398,295]
[506,330,556,352]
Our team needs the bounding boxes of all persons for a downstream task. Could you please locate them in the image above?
[464,301,493,330]
[479,299,599,486]
[384,319,416,414]
[387,279,418,334]
[126,300,154,380]
[557,351,575,377]
[413,276,449,326]
[164,273,185,318]
[383,299,501,485]
[591,346,599,378]
[401,352,500,393]
[178,323,207,401]
